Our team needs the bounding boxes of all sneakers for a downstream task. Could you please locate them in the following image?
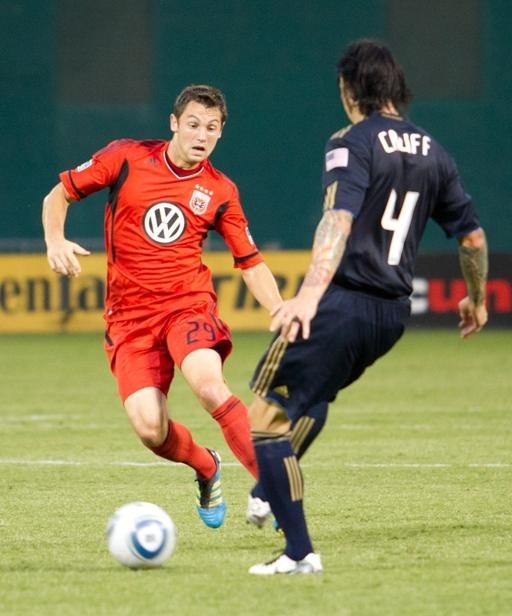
[245,496,272,527]
[193,448,226,528]
[248,550,323,576]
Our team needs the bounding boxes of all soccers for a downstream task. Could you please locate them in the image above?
[106,501,176,571]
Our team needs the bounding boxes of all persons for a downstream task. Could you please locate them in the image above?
[42,85,290,536]
[245,42,487,576]
